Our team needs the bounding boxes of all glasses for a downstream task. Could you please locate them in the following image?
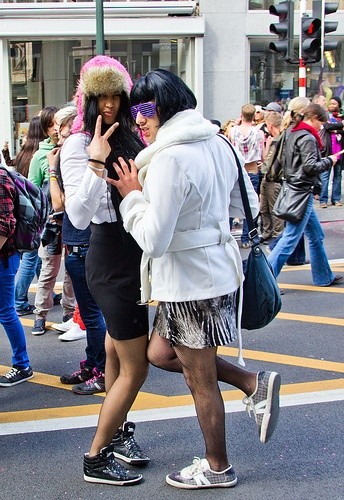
[130,101,156,121]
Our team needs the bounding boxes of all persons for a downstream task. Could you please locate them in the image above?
[17,117,62,316]
[52,105,87,341]
[46,113,103,395]
[27,106,76,335]
[60,56,150,485]
[224,95,344,267]
[105,68,281,489]
[0,165,34,387]
[268,104,343,295]
[2,129,30,166]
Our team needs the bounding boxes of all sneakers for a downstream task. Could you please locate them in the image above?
[111,421,151,465]
[0,365,33,387]
[242,371,281,445]
[60,360,96,384]
[72,367,105,395]
[82,445,142,485]
[166,457,238,489]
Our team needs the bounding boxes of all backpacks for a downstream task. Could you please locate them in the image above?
[0,165,48,253]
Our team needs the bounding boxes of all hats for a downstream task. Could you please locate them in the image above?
[70,56,149,148]
[261,102,282,114]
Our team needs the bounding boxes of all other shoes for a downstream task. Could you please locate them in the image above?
[61,313,73,323]
[332,201,342,206]
[332,275,344,284]
[31,319,45,334]
[58,323,86,341]
[51,318,75,332]
[53,293,62,306]
[321,203,327,208]
[16,304,35,316]
[314,195,319,200]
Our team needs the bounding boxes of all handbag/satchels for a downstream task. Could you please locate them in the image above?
[272,181,312,226]
[236,245,282,330]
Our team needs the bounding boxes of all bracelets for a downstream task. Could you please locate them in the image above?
[88,165,104,172]
[88,159,106,165]
[48,168,58,180]
